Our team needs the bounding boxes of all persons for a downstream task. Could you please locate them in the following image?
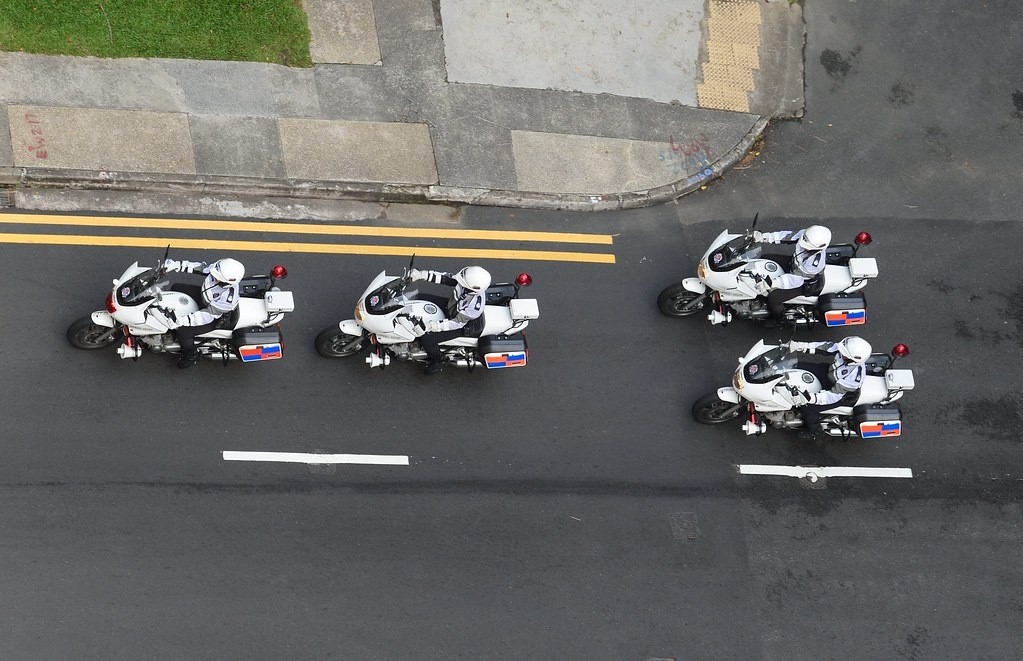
[754,224,833,328]
[784,337,872,439]
[160,258,246,369]
[407,265,492,376]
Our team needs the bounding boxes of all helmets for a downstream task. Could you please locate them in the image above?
[209,258,245,289]
[798,225,831,255]
[453,266,492,295]
[838,336,872,366]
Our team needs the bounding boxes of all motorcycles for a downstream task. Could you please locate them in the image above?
[657,212,879,327]
[67,244,294,362]
[691,338,915,439]
[314,252,540,370]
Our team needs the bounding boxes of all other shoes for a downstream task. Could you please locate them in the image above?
[765,320,782,328]
[797,428,822,440]
[177,352,198,368]
[424,362,443,376]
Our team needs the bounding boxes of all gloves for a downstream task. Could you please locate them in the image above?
[169,315,190,330]
[412,320,441,337]
[749,231,770,242]
[780,340,806,353]
[161,259,181,273]
[792,392,816,408]
[407,269,428,281]
[756,277,779,296]
